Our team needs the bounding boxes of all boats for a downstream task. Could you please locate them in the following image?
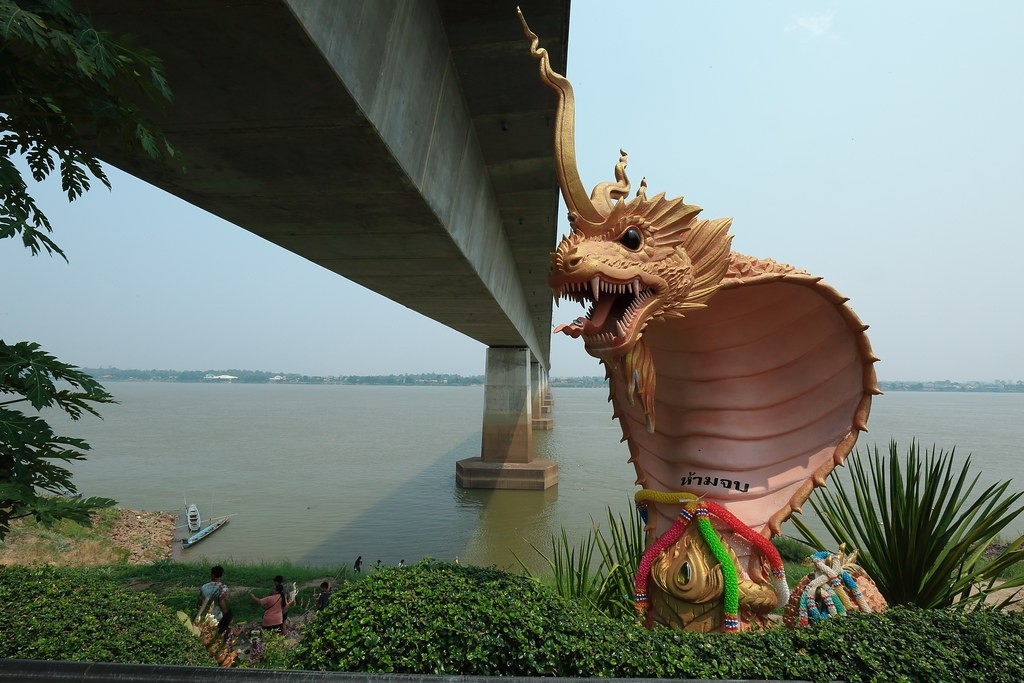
[180,518,229,550]
[184,503,201,533]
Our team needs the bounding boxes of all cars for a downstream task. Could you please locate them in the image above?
[204,373,215,380]
[102,374,112,381]
[269,375,283,381]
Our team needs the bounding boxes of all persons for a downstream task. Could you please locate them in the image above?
[376,560,381,566]
[273,575,291,622]
[313,582,331,609]
[195,565,228,621]
[354,556,362,573]
[248,585,294,633]
[398,559,404,567]
